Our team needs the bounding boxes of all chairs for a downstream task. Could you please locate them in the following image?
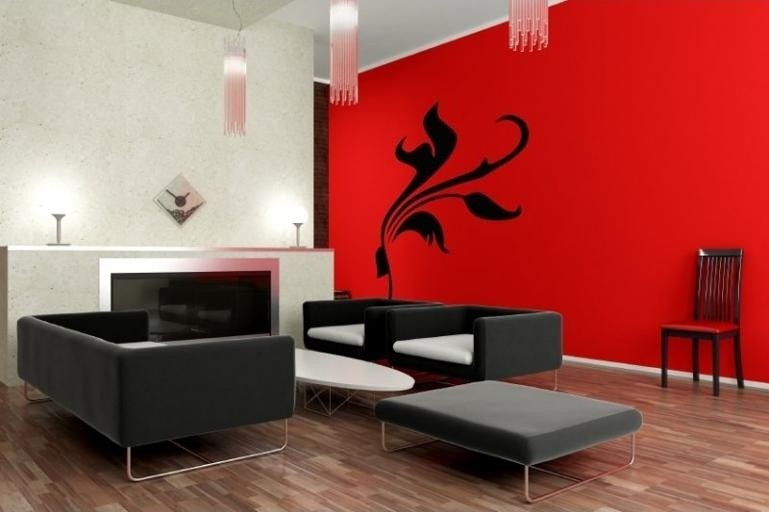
[303,298,561,393]
[660,246,745,398]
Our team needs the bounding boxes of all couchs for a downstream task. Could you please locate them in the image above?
[17,310,295,484]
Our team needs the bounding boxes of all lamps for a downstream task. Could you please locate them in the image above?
[224,0,361,138]
[509,0,548,53]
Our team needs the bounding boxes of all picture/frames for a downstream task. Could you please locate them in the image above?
[153,173,208,228]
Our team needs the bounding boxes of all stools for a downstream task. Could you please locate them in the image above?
[373,379,644,506]
